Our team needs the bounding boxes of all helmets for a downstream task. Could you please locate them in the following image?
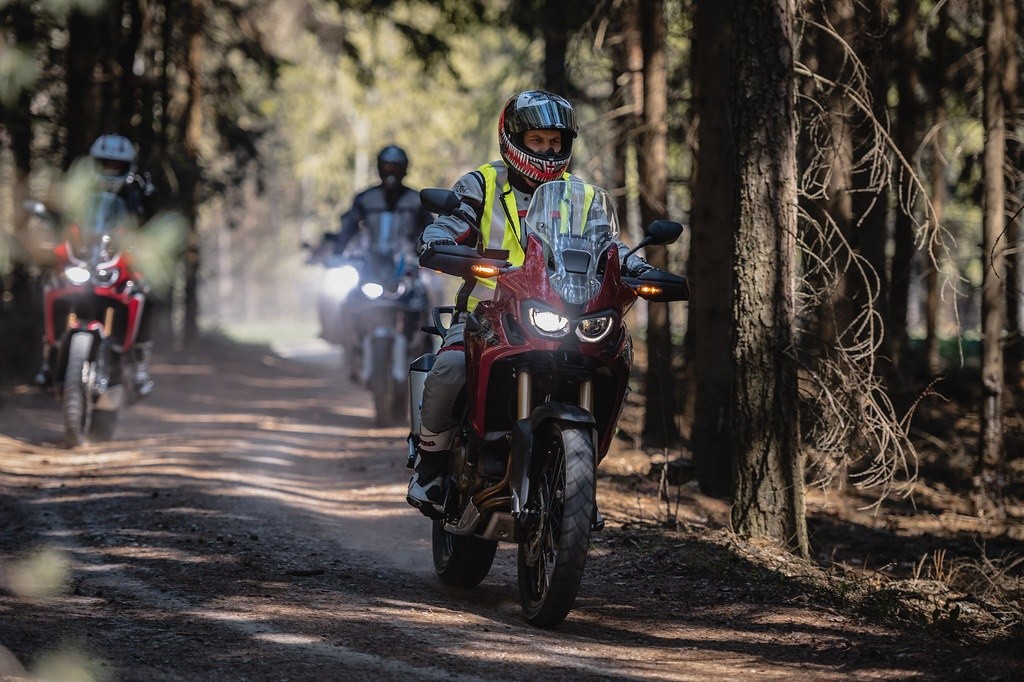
[89,136,138,194]
[498,90,578,182]
[377,146,408,190]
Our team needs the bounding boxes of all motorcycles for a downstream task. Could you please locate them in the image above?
[411,180,689,626]
[296,232,433,427]
[20,190,157,448]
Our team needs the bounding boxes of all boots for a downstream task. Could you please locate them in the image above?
[36,337,56,388]
[133,339,154,398]
[348,339,364,383]
[408,422,455,505]
[590,506,605,530]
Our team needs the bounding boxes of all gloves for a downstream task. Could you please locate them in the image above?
[637,267,664,278]
[420,238,458,258]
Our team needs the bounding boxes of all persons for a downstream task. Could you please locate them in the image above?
[335,146,434,390]
[404,89,668,519]
[35,134,159,397]
